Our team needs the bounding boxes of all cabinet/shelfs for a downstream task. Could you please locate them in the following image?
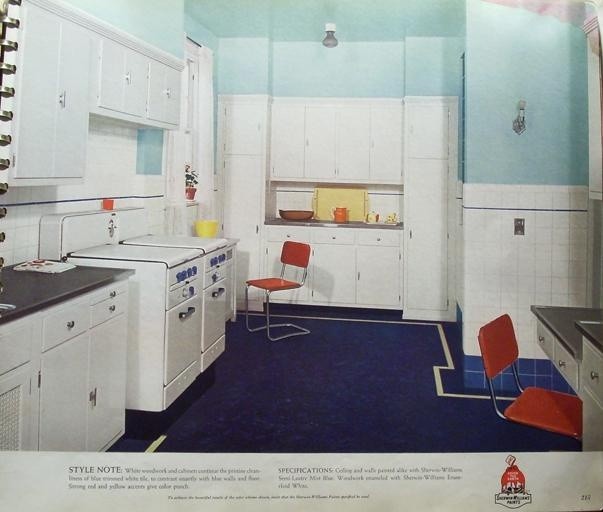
[0,325,32,451]
[96,37,149,117]
[216,94,459,323]
[10,4,91,180]
[201,247,225,374]
[126,259,203,413]
[147,59,181,126]
[533,321,579,396]
[579,336,603,452]
[36,282,128,452]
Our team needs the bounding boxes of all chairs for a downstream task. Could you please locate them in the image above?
[244,240,311,342]
[477,312,583,442]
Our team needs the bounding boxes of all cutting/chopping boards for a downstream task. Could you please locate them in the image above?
[311,186,369,222]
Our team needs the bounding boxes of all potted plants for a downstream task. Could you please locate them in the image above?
[185,164,198,200]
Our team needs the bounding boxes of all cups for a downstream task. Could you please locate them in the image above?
[102,199,114,211]
[333,207,347,224]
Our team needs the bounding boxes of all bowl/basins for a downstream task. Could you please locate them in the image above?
[277,209,314,221]
